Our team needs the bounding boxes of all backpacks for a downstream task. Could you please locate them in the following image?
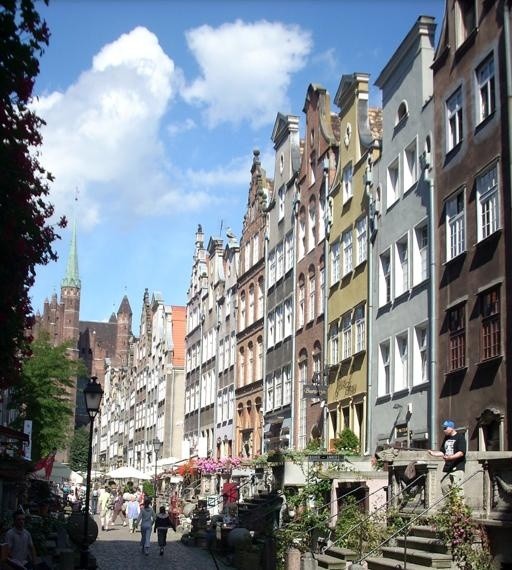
[104,495,115,510]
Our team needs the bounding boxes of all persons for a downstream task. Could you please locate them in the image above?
[61,484,144,534]
[5,511,36,570]
[428,420,466,510]
[135,497,156,556]
[154,507,177,556]
[0,543,9,560]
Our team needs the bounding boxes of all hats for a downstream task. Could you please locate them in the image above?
[441,420,455,428]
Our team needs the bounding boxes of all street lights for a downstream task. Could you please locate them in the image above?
[80,375,104,570]
[152,436,161,514]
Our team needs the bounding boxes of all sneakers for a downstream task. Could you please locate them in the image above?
[160,548,164,555]
